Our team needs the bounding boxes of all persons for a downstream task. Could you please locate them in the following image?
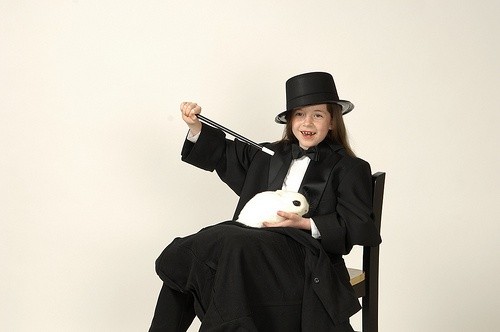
[145,70,375,332]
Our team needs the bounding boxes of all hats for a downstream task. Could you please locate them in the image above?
[274,71,354,126]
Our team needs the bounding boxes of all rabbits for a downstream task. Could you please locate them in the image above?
[235,189,309,228]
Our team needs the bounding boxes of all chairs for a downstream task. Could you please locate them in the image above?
[230,171,387,331]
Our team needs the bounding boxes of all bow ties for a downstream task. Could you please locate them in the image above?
[290,143,320,162]
